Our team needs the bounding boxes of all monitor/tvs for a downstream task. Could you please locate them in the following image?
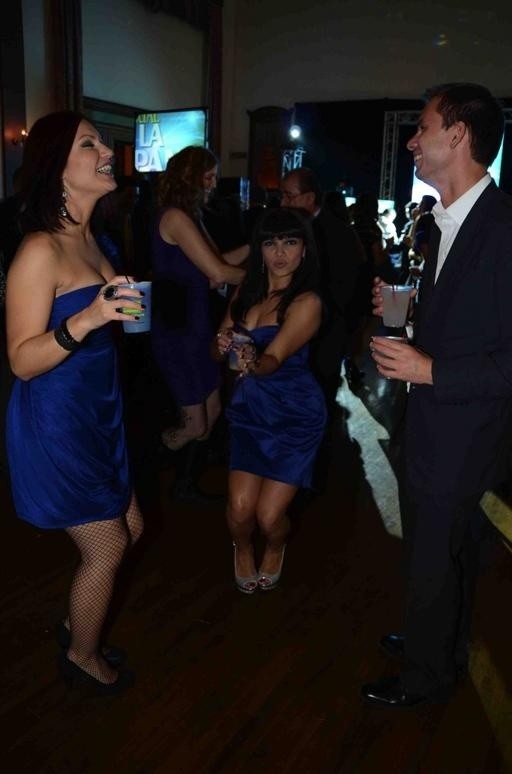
[131,105,210,176]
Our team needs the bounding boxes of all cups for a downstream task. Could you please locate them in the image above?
[228,334,254,373]
[379,286,415,328]
[371,335,409,379]
[117,280,152,334]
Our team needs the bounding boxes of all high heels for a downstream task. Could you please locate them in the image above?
[58,619,134,693]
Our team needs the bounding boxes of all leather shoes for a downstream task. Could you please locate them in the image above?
[359,634,470,709]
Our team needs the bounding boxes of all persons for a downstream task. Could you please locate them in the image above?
[208,204,329,598]
[6,108,147,688]
[146,144,253,506]
[362,77,511,707]
[279,167,372,447]
[0,163,439,281]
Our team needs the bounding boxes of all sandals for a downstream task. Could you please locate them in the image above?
[234,541,285,594]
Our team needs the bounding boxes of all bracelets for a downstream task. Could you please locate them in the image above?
[54,316,80,353]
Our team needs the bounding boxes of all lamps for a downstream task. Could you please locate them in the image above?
[287,106,304,143]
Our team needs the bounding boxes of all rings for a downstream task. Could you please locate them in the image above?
[112,289,119,297]
[216,333,222,337]
